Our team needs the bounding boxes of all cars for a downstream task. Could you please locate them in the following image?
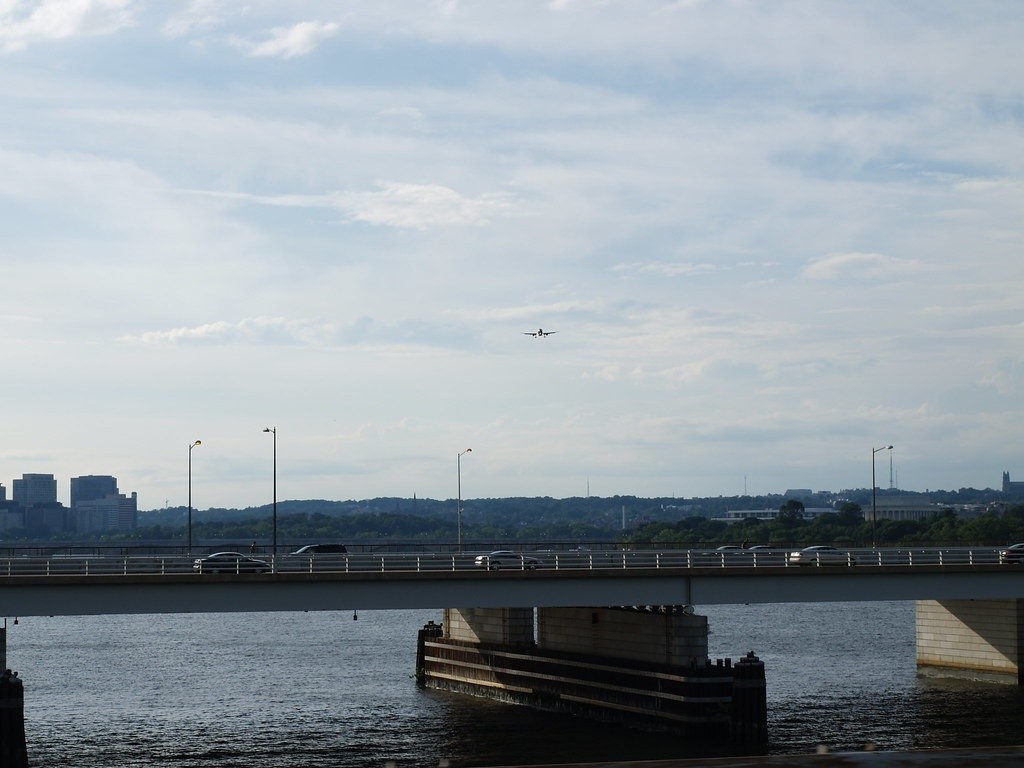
[746,546,779,555]
[788,545,856,567]
[192,551,272,574]
[702,545,746,556]
[1001,544,1024,565]
[474,550,543,570]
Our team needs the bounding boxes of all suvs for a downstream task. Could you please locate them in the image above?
[282,544,349,572]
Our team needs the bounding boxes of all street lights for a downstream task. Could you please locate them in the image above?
[188,440,201,548]
[458,449,472,554]
[262,427,277,556]
[872,444,892,546]
